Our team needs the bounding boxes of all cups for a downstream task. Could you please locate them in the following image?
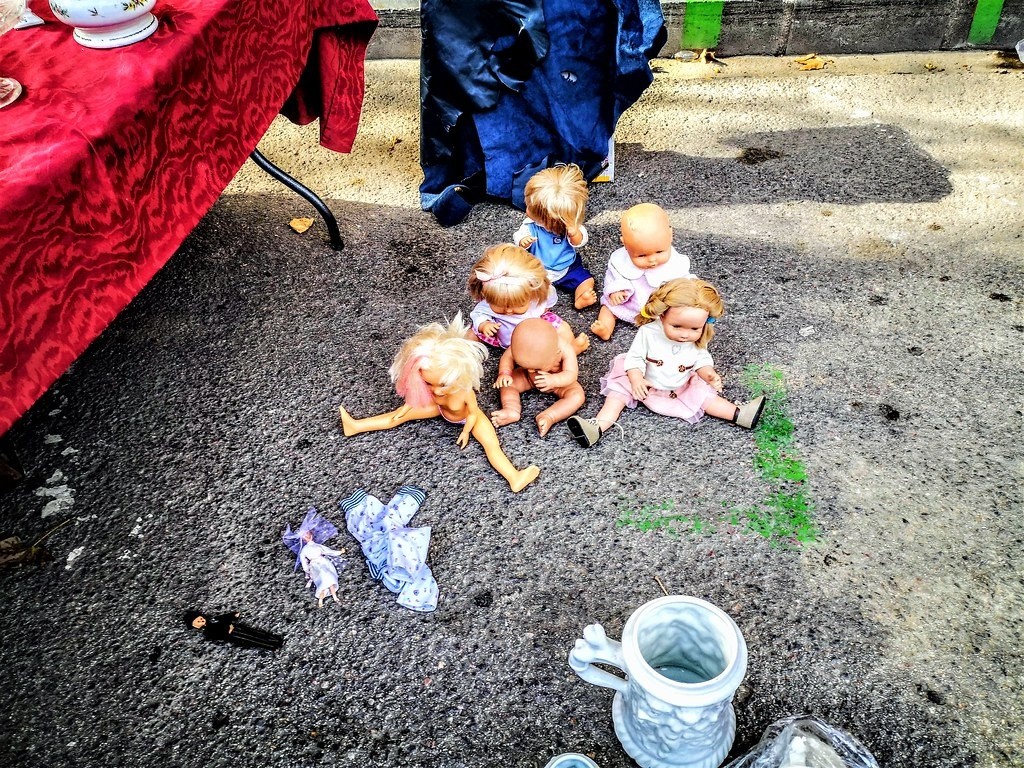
[569,595,748,768]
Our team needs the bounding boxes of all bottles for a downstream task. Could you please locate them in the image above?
[49,0,159,49]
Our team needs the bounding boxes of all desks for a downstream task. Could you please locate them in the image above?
[0,0,382,431]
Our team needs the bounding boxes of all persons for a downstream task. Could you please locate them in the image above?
[568,276,766,449]
[339,308,539,493]
[589,202,698,341]
[281,508,345,607]
[491,317,585,438]
[467,243,590,358]
[513,165,597,310]
[182,611,284,651]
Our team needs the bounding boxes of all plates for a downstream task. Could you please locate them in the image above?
[0,78,22,109]
